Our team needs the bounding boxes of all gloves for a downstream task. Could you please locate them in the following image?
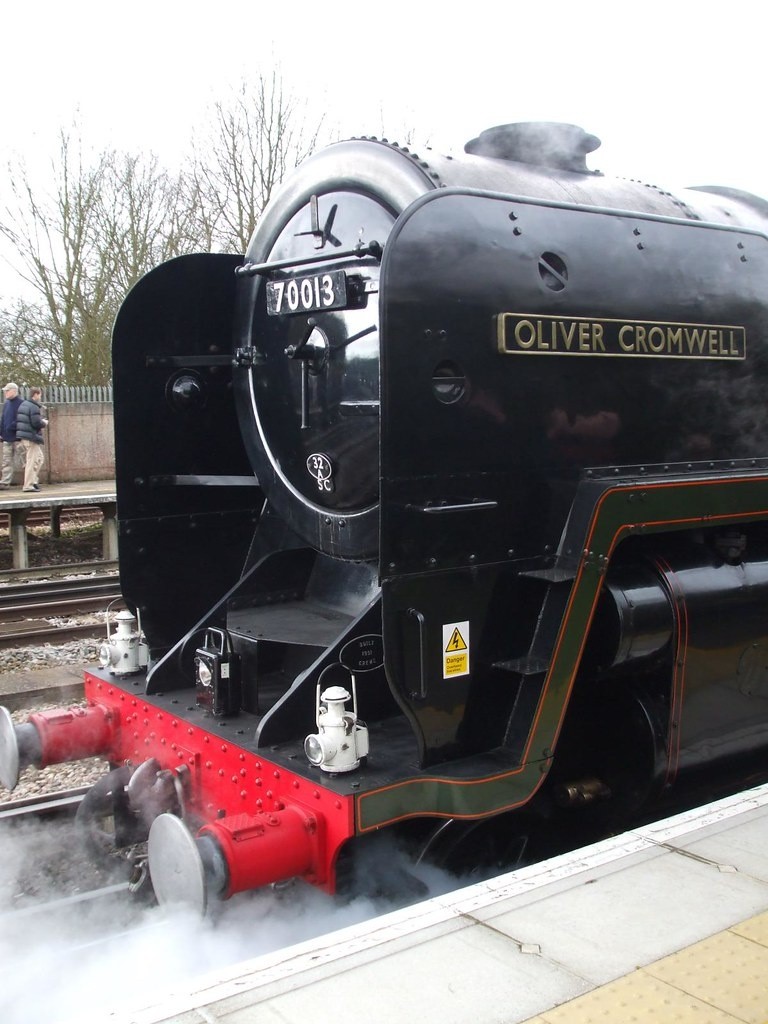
[42,418,48,425]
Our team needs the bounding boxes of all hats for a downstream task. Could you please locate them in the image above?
[2,383,18,391]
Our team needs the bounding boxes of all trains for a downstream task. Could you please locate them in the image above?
[0,123,768,938]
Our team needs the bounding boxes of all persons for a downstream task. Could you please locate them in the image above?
[0,383,26,490]
[16,387,49,491]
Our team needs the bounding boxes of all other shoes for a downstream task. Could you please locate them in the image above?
[0,483,11,490]
[22,484,41,492]
